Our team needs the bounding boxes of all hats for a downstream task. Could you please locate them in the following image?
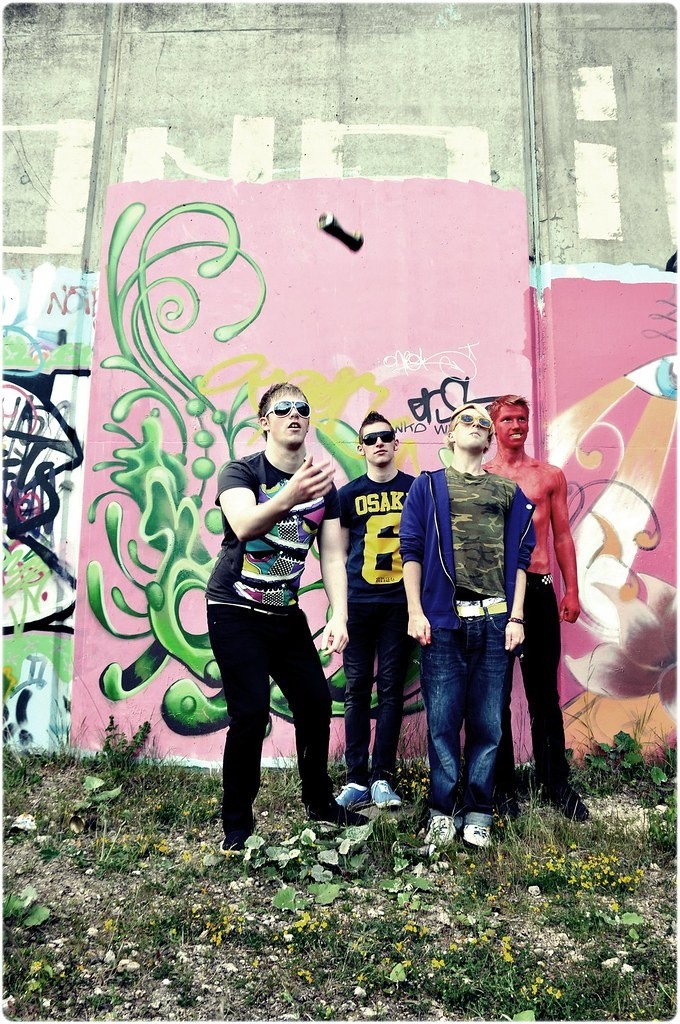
[450,403,493,425]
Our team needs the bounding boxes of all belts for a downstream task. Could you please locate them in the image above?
[457,601,507,617]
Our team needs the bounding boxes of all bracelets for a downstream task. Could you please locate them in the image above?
[508,617,526,625]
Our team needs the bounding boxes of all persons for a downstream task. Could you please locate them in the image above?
[205,383,369,855]
[399,402,537,847]
[479,393,593,824]
[335,412,415,813]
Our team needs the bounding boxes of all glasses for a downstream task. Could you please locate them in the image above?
[452,415,494,429]
[265,399,311,418]
[361,431,396,446]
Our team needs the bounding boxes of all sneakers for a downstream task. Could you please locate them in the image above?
[462,824,490,848]
[493,791,519,822]
[424,815,458,846]
[219,819,254,855]
[335,782,372,811]
[535,779,590,820]
[370,779,402,807]
[307,802,369,826]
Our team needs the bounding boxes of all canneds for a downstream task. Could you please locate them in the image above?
[318,213,365,253]
